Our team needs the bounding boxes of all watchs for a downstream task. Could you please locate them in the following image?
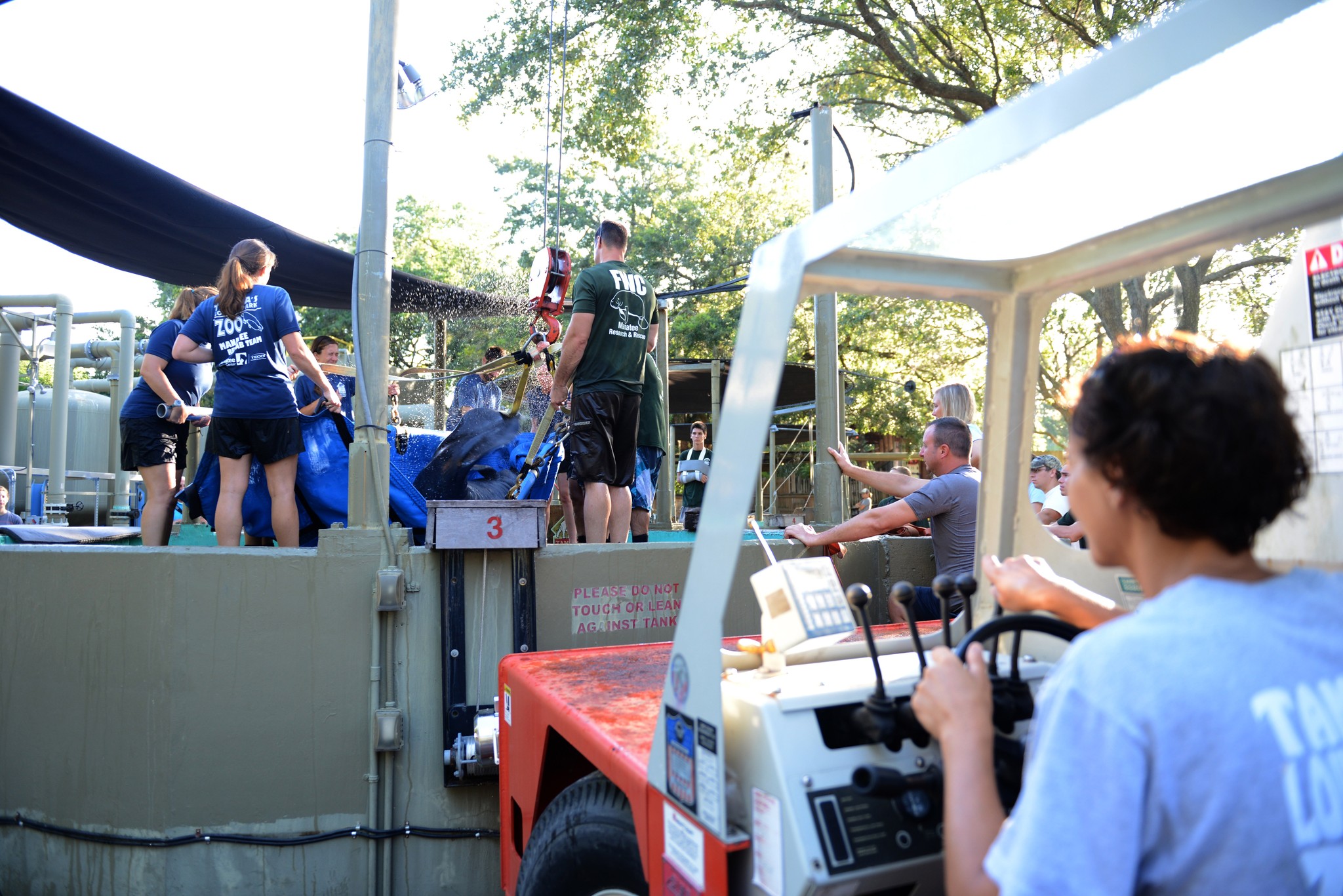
[168,400,184,409]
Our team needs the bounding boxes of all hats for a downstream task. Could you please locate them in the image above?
[1031,454,1063,472]
[859,488,870,494]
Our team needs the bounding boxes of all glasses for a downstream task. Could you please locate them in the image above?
[1032,468,1053,473]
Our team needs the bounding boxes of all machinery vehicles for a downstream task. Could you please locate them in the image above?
[422,0,1343,896]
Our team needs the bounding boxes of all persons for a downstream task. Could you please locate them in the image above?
[528,364,583,545]
[0,486,24,525]
[932,381,984,470]
[447,344,503,432]
[855,488,872,514]
[1047,466,1089,549]
[783,323,1343,896]
[676,421,713,529]
[115,220,666,547]
